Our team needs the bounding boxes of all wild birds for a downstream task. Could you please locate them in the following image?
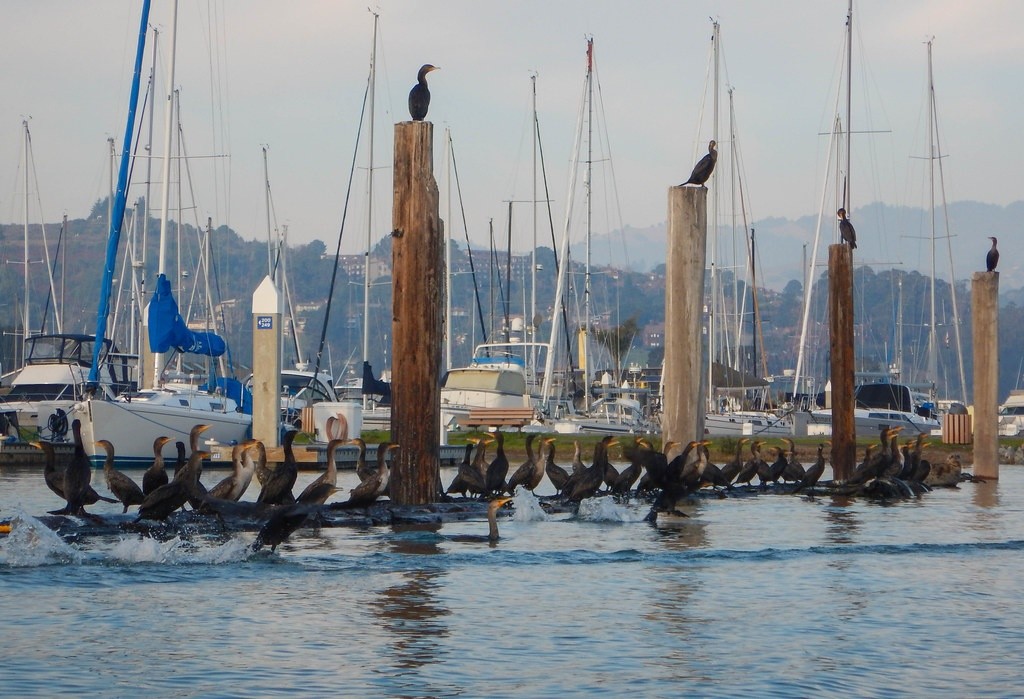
[986,236,999,272]
[679,140,718,188]
[837,207,857,250]
[408,64,441,121]
[26,412,963,554]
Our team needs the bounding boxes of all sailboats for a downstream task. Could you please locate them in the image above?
[2,0,1024,473]
